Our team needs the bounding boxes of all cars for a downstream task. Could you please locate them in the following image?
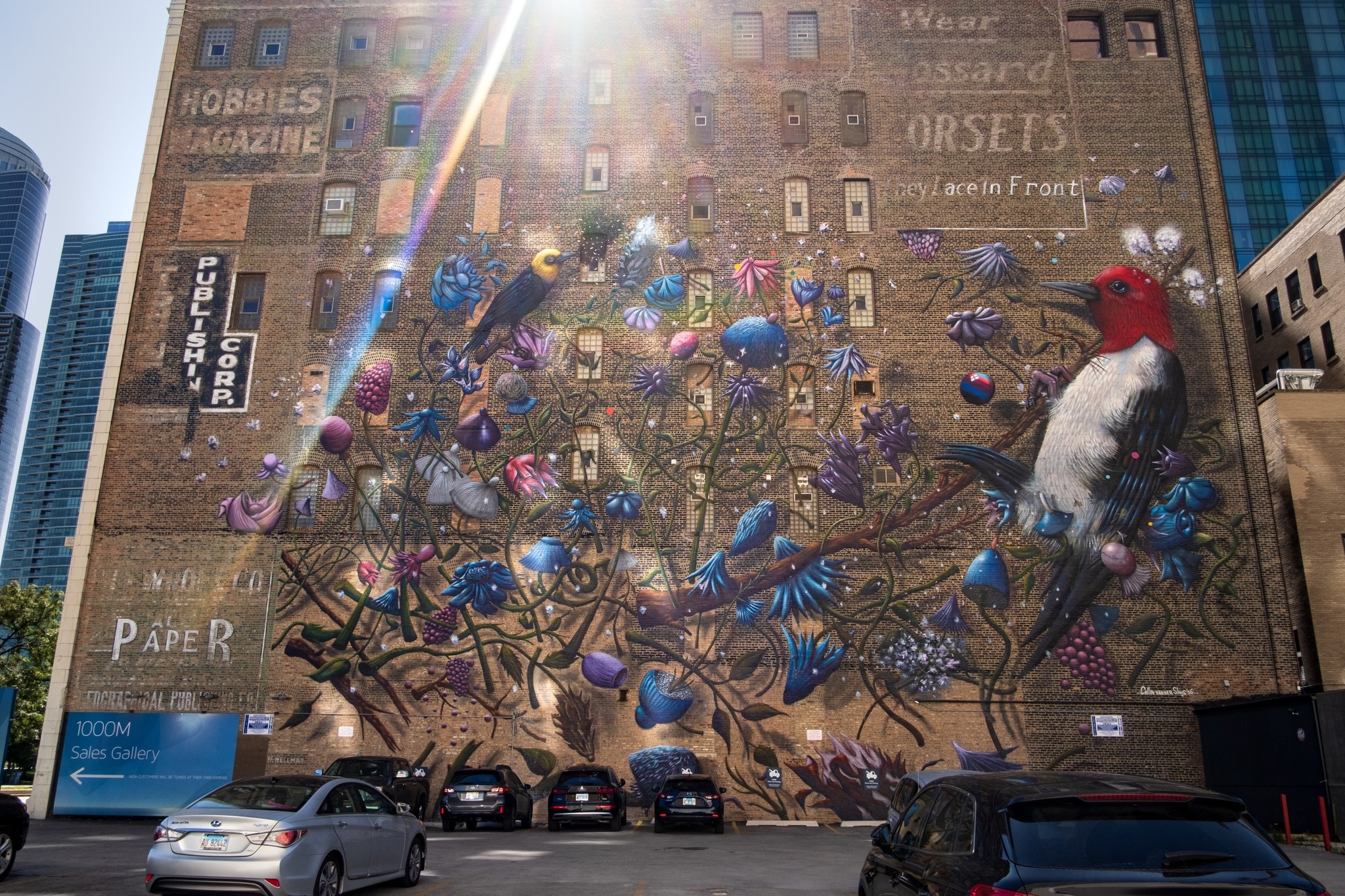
[144,773,429,896]
[857,771,1337,896]
[653,769,727,835]
[0,792,31,884]
[886,769,986,832]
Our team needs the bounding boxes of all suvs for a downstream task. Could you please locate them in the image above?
[439,764,533,833]
[547,764,627,833]
[314,755,430,823]
